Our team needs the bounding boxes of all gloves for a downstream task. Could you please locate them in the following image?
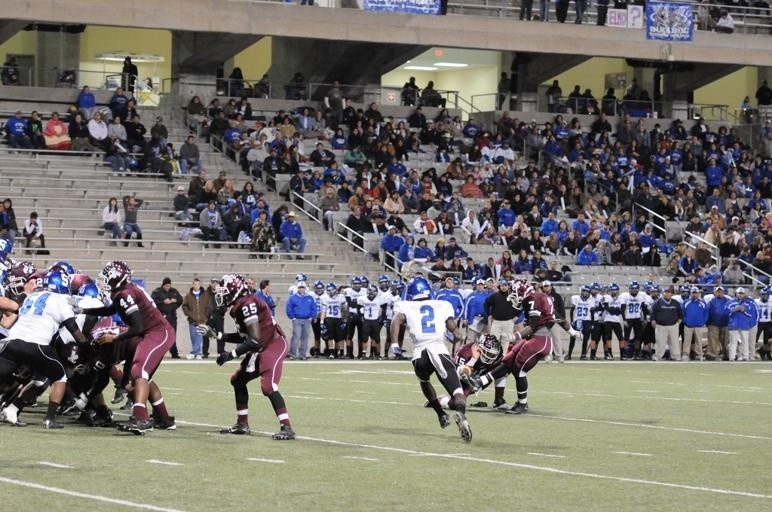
[338,322,349,331]
[195,322,223,341]
[215,350,235,367]
[387,343,405,360]
[319,324,328,336]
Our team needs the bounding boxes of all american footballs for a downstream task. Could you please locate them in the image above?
[455,364,473,382]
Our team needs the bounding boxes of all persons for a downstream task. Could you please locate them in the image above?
[0,261,176,434]
[0,58,771,362]
[196,274,296,441]
[390,278,554,442]
[520,0,735,30]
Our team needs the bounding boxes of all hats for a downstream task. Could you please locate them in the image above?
[645,222,654,229]
[715,285,725,292]
[736,287,746,293]
[211,278,220,286]
[286,212,302,218]
[673,118,684,125]
[498,279,508,286]
[161,277,173,285]
[542,279,551,287]
[388,225,397,232]
[663,286,673,292]
[297,281,308,288]
[485,276,496,283]
[710,205,719,209]
[476,278,486,285]
[449,236,457,242]
[690,287,700,292]
[437,237,446,243]
[731,215,739,220]
[177,186,185,192]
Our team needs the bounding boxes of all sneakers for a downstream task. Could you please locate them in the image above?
[327,351,335,359]
[492,398,512,411]
[604,354,614,361]
[423,395,441,407]
[271,427,297,440]
[591,354,600,360]
[87,412,114,427]
[346,353,354,360]
[643,352,650,361]
[3,403,29,427]
[619,352,627,361]
[203,353,209,358]
[111,385,125,403]
[151,414,177,430]
[42,416,66,430]
[56,396,78,415]
[74,407,98,425]
[196,353,203,361]
[120,392,135,410]
[437,414,451,429]
[463,372,483,392]
[631,352,641,362]
[374,352,383,360]
[580,354,588,361]
[312,350,320,358]
[186,352,195,360]
[505,401,530,414]
[220,423,252,435]
[338,353,345,358]
[116,416,155,436]
[564,354,572,361]
[452,410,473,444]
[359,352,368,360]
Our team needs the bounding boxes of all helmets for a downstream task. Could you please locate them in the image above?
[78,283,99,298]
[758,288,769,294]
[361,276,368,283]
[514,280,533,297]
[580,284,590,292]
[54,261,75,274]
[47,272,70,294]
[395,283,405,289]
[590,283,601,290]
[368,283,379,292]
[609,283,620,290]
[16,261,37,278]
[679,284,689,291]
[351,276,361,284]
[102,260,130,282]
[477,332,502,364]
[314,280,324,289]
[219,272,249,305]
[630,281,639,288]
[453,277,462,290]
[649,286,661,292]
[1,236,15,257]
[378,274,389,282]
[408,276,431,299]
[600,284,608,294]
[326,283,336,290]
[391,279,401,285]
[295,273,308,282]
[71,274,92,291]
